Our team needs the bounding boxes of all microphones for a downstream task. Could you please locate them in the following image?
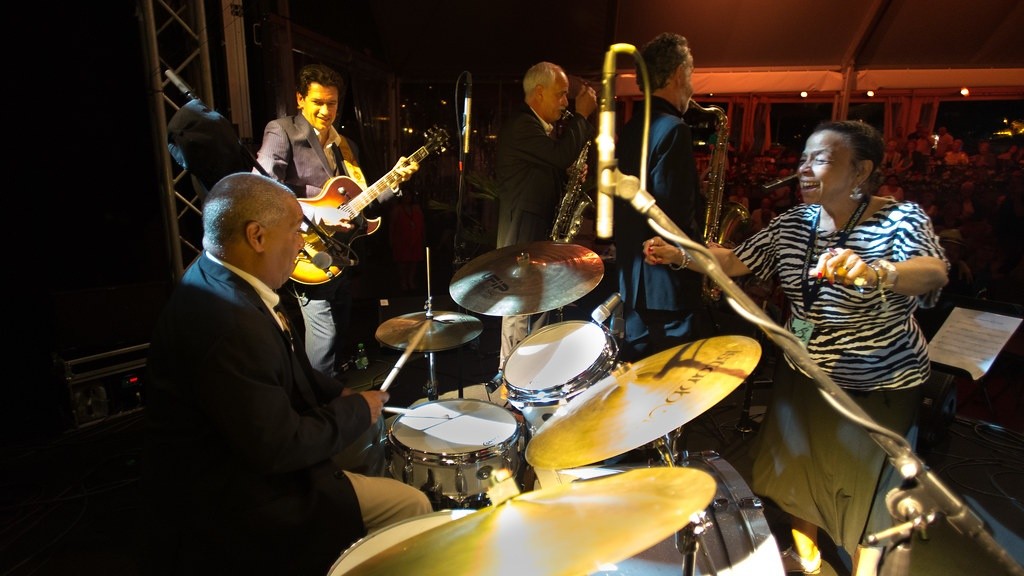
[485,370,504,394]
[313,251,357,270]
[590,294,622,324]
[462,73,472,153]
[595,48,617,239]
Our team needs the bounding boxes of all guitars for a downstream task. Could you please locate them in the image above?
[288,123,452,286]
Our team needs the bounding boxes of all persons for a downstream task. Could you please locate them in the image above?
[252,62,381,395]
[642,120,950,576]
[615,30,726,362]
[492,60,597,249]
[723,121,1023,293]
[391,185,427,295]
[149,172,434,575]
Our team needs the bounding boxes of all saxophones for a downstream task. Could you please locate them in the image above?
[689,97,753,308]
[548,108,593,243]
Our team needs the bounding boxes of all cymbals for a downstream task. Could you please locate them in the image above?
[373,311,484,353]
[524,334,764,471]
[448,240,605,318]
[341,465,718,576]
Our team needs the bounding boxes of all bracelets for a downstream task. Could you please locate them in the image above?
[854,265,882,294]
[669,247,693,270]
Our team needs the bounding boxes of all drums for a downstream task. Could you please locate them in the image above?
[385,398,527,502]
[326,508,478,575]
[589,450,787,576]
[498,319,626,433]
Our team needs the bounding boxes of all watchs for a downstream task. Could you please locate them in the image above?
[872,259,899,294]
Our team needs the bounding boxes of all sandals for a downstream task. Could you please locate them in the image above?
[780,546,822,575]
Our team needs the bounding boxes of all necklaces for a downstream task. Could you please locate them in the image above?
[406,206,413,212]
[811,225,842,262]
